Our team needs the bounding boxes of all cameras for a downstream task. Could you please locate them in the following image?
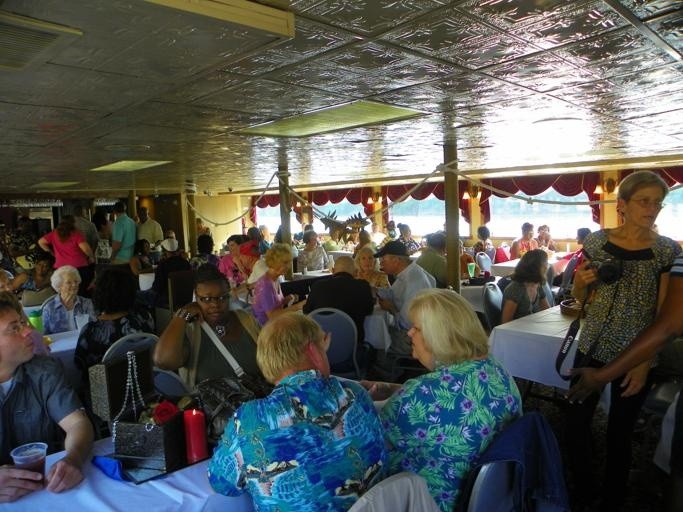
[587,255,623,284]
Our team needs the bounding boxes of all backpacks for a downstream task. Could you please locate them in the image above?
[191,376,271,447]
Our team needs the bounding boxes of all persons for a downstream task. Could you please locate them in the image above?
[563,250,683,405]
[0,201,593,512]
[558,170,683,512]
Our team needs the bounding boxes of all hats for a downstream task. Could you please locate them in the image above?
[239,238,261,258]
[373,240,410,259]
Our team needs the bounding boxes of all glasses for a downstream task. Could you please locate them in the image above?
[10,318,31,335]
[631,197,663,210]
[195,291,230,304]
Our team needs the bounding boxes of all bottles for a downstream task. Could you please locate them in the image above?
[27,310,44,335]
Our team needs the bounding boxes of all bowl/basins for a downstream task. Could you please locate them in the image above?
[559,299,582,316]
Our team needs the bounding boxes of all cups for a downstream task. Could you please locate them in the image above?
[467,263,477,280]
[327,261,334,273]
[74,314,89,334]
[9,441,49,489]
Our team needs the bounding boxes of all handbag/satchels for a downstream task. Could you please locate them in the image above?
[21,285,54,306]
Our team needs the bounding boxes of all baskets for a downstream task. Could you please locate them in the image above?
[559,297,585,320]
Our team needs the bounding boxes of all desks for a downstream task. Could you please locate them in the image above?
[0,244,683,512]
